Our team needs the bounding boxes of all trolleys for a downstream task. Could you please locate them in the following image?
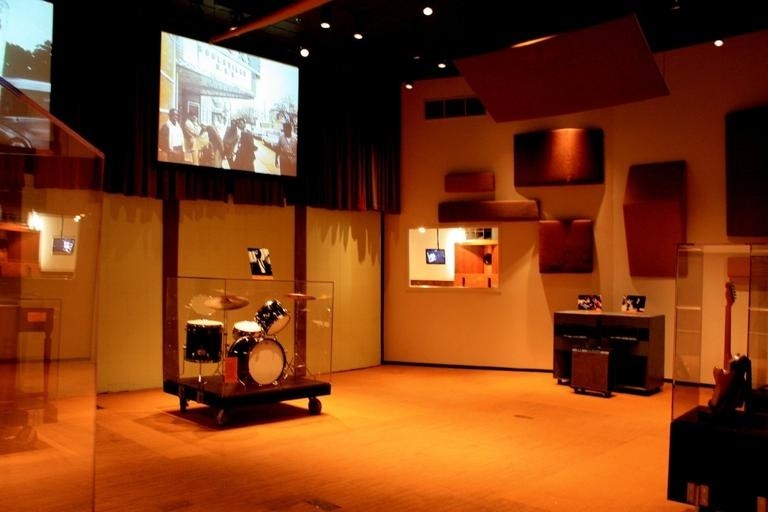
[160,273,334,426]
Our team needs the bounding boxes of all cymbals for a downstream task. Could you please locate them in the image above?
[204,294,248,310]
[284,292,316,301]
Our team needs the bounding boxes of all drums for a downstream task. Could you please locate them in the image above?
[232,321,263,341]
[228,337,286,387]
[185,319,223,364]
[254,298,290,335]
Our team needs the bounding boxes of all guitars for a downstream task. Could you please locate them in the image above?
[709,279,746,413]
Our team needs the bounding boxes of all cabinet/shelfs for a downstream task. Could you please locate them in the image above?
[668,240,768,512]
[0,70,103,512]
[553,310,664,392]
[164,273,335,426]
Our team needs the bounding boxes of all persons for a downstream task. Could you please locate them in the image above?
[274,121,296,176]
[158,104,258,175]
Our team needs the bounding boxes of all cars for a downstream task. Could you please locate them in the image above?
[0,75,52,150]
[246,117,297,151]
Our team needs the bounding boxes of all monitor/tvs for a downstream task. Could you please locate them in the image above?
[51,238,76,254]
[425,249,447,266]
